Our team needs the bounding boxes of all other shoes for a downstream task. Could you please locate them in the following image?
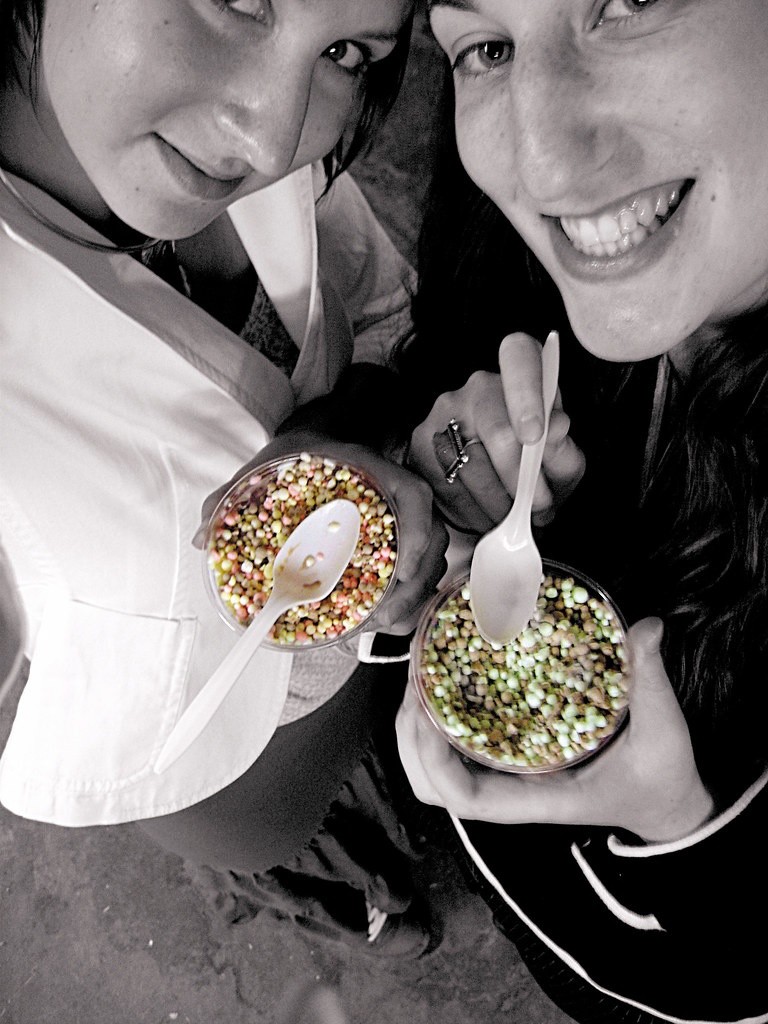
[366,900,431,962]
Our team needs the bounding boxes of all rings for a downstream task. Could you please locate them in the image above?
[432,417,483,485]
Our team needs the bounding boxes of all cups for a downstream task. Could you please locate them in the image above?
[409,558,635,774]
[202,453,403,652]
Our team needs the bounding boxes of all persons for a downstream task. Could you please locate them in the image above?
[372,0,768,1024]
[0,0,459,961]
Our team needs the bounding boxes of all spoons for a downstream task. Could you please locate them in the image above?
[156,498,361,774]
[470,330,561,647]
